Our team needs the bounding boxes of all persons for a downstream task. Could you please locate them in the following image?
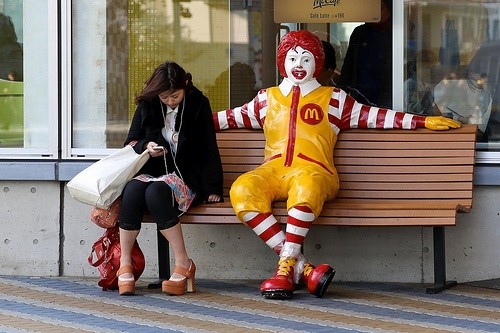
[405,41,500,141]
[339,0,392,107]
[212,30,462,299]
[116,61,223,296]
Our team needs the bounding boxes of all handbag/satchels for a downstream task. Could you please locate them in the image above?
[66,139,152,209]
[88,223,145,289]
[89,198,121,230]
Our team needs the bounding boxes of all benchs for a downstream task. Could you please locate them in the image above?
[141,124,479,294]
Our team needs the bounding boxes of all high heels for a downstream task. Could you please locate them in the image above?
[116,266,135,294]
[161,259,196,295]
